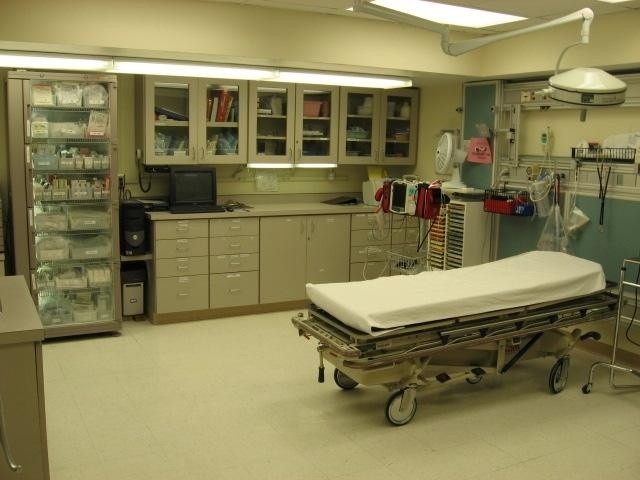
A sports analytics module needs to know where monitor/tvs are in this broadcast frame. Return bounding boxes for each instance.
[170,168,216,206]
[389,180,415,215]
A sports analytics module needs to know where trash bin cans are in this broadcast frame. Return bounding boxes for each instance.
[122,268,145,318]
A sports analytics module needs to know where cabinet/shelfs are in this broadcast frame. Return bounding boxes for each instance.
[351,215,420,281]
[4,70,122,339]
[260,213,349,314]
[144,218,260,325]
[135,74,419,165]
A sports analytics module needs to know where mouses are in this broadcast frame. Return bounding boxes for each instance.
[227,207,233,211]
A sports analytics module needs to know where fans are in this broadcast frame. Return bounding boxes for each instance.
[433,133,468,190]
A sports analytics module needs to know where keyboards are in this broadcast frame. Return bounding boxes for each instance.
[172,205,225,214]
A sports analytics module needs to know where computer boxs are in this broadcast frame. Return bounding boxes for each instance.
[119,199,146,256]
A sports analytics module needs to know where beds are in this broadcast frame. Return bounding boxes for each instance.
[290,253,626,426]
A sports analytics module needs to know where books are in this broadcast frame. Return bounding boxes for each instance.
[155,105,184,120]
[206,91,238,122]
